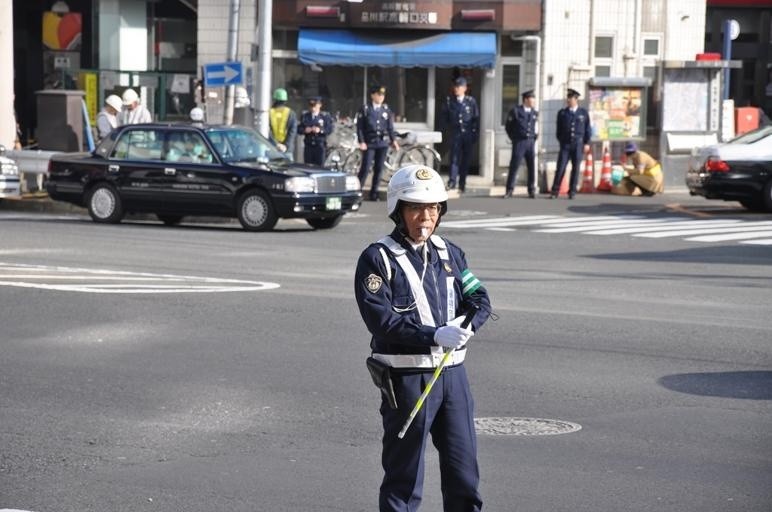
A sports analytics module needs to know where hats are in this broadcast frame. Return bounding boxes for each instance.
[43,122,364,232]
[0,155,22,201]
[686,122,772,213]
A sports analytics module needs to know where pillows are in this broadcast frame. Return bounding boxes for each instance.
[104,88,139,113]
[386,164,451,234]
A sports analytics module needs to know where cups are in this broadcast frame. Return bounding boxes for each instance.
[404,203,444,216]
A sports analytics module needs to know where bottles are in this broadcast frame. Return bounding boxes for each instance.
[625,143,637,154]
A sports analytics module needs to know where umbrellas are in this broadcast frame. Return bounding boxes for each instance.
[504,187,574,199]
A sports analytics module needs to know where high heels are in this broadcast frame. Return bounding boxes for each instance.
[557,169,569,196]
[595,147,615,191]
[578,150,597,194]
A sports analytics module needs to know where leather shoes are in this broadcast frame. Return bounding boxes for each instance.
[434,326,475,351]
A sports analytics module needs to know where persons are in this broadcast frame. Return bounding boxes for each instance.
[503,87,542,198]
[299,96,333,167]
[95,93,124,139]
[547,88,591,201]
[440,77,479,192]
[269,89,298,165]
[353,163,496,511]
[121,87,162,149]
[621,142,667,198]
[357,83,401,203]
